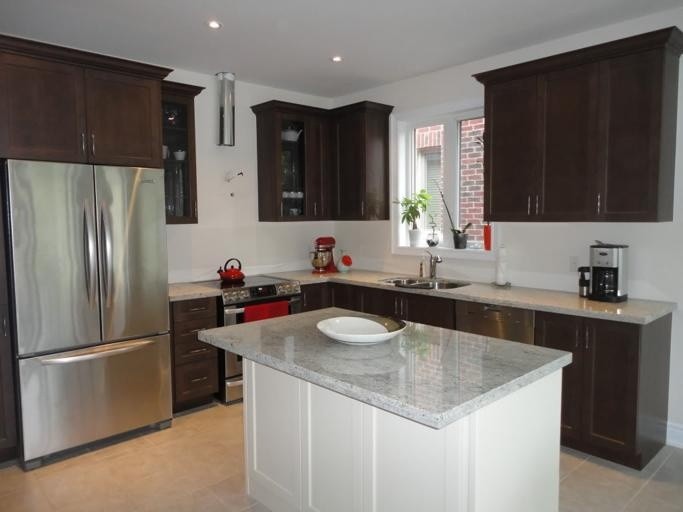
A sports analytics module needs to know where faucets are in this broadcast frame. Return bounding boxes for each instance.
[425,250,442,280]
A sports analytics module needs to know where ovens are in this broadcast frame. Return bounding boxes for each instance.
[219,294,301,404]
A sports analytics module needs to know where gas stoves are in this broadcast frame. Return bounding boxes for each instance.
[191,274,301,304]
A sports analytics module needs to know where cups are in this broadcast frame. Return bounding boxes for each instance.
[576,267,590,297]
[281,191,304,199]
[161,145,170,160]
[172,150,186,160]
[579,296,588,311]
[288,208,301,216]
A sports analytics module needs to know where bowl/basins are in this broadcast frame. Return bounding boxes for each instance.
[317,315,406,346]
[310,342,407,374]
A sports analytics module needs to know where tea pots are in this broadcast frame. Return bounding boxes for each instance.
[216,258,244,281]
[219,278,244,288]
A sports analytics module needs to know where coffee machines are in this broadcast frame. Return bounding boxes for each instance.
[587,240,629,304]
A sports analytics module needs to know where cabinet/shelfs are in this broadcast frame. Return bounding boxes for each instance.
[250,100,393,222]
[170,280,330,419]
[472,26,683,223]
[161,79,206,224]
[0,34,174,169]
[330,280,679,469]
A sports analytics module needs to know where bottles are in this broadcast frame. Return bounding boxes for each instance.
[483,221,490,251]
[335,248,350,273]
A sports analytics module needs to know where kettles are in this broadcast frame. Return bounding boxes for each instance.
[280,126,303,141]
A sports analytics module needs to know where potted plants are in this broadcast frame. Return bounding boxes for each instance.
[432,178,472,249]
[392,189,432,248]
[426,214,439,248]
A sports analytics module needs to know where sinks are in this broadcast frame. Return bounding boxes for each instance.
[377,276,426,287]
[409,279,473,290]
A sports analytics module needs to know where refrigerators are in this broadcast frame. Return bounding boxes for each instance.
[0,156,175,471]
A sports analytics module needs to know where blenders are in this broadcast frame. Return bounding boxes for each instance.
[307,236,338,273]
